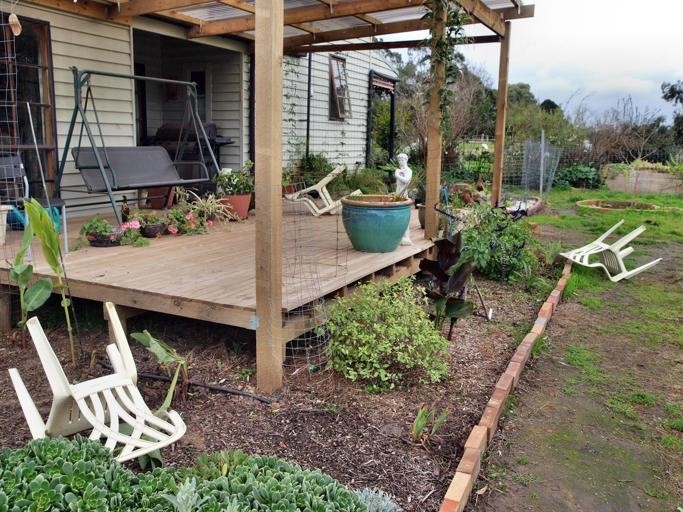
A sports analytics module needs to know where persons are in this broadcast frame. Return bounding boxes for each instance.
[394,153,413,198]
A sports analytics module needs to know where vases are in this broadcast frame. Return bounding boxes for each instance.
[220,191,251,220]
[341,193,412,254]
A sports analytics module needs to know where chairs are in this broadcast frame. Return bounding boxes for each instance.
[284,165,362,217]
[6,298,188,463]
[1,155,68,267]
[555,217,663,283]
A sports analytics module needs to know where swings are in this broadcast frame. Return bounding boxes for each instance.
[71,80,210,194]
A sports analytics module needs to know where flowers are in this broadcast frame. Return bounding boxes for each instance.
[69,199,215,252]
[214,161,256,195]
